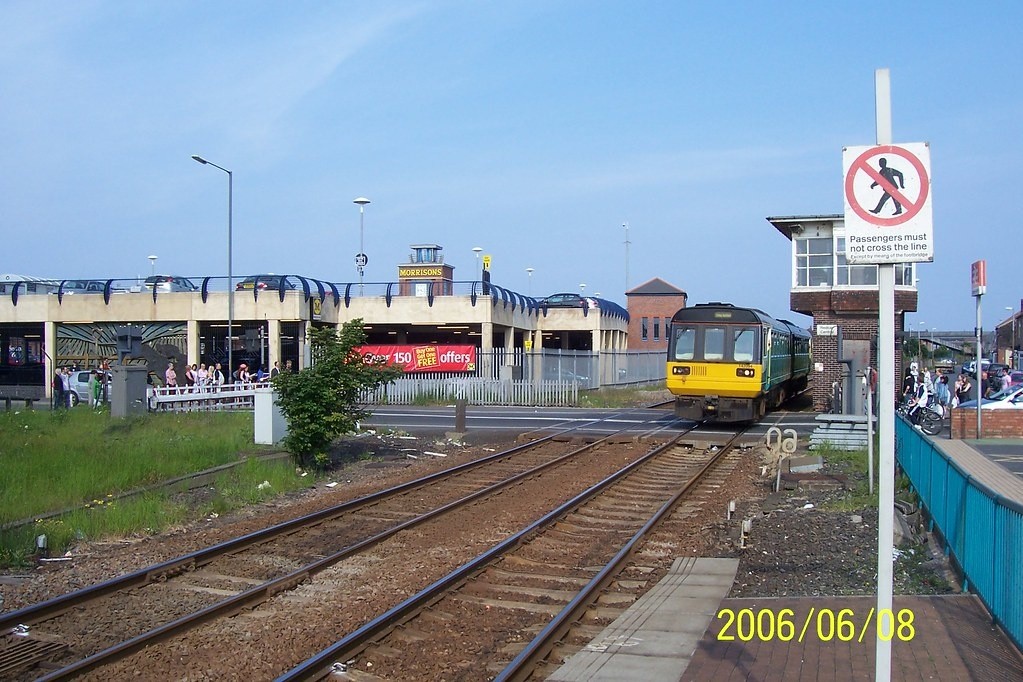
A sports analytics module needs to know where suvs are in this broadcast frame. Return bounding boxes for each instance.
[536,293,587,308]
[64,370,154,407]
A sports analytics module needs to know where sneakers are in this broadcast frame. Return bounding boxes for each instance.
[914,424,921,430]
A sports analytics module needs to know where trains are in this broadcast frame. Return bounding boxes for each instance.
[665,301,812,424]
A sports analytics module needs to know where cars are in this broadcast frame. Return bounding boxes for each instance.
[1007,370,1023,393]
[962,360,990,378]
[236,274,296,291]
[582,297,598,308]
[49,280,111,294]
[144,274,199,293]
[987,362,1009,381]
[957,383,1023,409]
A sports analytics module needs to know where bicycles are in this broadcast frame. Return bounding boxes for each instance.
[898,394,945,436]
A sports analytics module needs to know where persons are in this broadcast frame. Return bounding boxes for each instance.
[871,368,877,414]
[1001,369,1011,396]
[982,373,990,398]
[184,363,224,410]
[166,362,177,395]
[53,366,73,410]
[903,366,971,431]
[233,360,293,407]
[90,357,112,409]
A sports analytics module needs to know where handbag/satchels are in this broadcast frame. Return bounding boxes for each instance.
[951,397,959,409]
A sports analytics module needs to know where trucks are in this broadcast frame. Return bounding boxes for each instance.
[935,360,954,374]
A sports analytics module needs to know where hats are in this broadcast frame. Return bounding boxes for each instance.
[240,364,246,368]
[73,361,77,363]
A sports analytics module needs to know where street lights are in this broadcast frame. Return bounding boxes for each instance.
[190,155,234,403]
[580,284,586,297]
[472,246,482,295]
[353,197,370,296]
[931,328,937,358]
[919,322,925,362]
[1005,306,1015,351]
[526,267,534,297]
